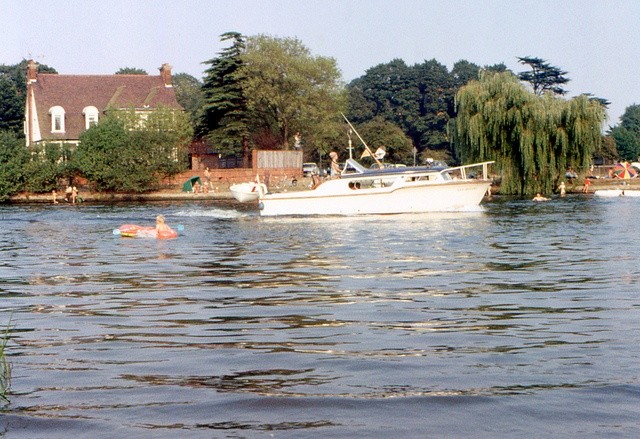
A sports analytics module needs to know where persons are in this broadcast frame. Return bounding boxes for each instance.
[294,129,302,149]
[608,168,621,179]
[633,170,640,178]
[349,183,355,190]
[65,185,80,204]
[192,167,212,196]
[154,214,171,234]
[636,171,640,178]
[356,183,360,189]
[329,152,342,176]
[583,178,589,194]
[587,169,598,178]
[532,193,549,201]
[52,190,58,204]
[564,167,578,179]
[559,181,565,195]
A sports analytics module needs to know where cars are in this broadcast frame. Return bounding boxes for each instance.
[468,172,501,187]
[302,163,320,176]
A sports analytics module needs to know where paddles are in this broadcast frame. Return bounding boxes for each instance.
[169,225,184,231]
[113,227,138,236]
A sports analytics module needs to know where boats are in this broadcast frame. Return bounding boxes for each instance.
[258,159,495,216]
[595,189,640,197]
[231,184,267,201]
